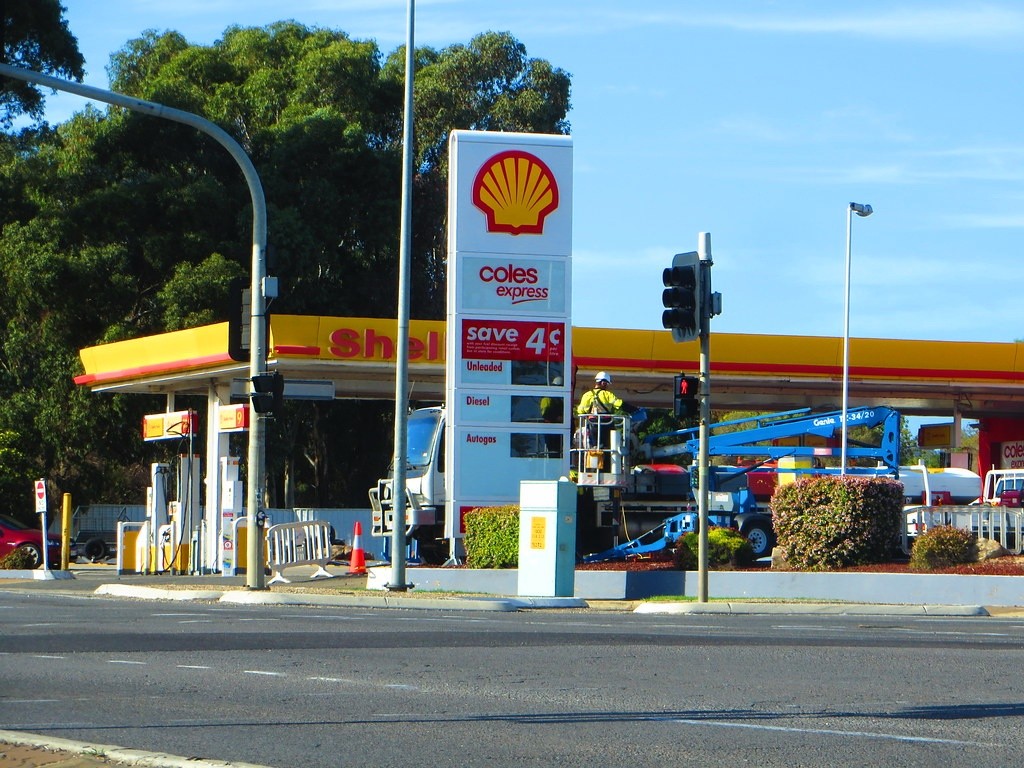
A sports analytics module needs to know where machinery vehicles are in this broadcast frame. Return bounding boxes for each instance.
[368,401,902,567]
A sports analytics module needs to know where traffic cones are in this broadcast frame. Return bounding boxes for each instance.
[344,521,369,574]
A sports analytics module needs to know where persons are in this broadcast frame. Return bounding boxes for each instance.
[577,371,640,472]
[540,377,564,458]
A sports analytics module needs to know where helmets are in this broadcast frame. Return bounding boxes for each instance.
[595,371,613,385]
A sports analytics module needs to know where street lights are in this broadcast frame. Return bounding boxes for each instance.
[841,201,874,477]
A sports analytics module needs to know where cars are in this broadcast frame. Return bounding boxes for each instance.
[0,514,78,570]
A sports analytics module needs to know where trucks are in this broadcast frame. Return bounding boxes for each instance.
[899,458,1024,553]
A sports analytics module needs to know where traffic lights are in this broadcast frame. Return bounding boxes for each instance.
[673,374,700,420]
[250,373,285,414]
[662,250,702,342]
